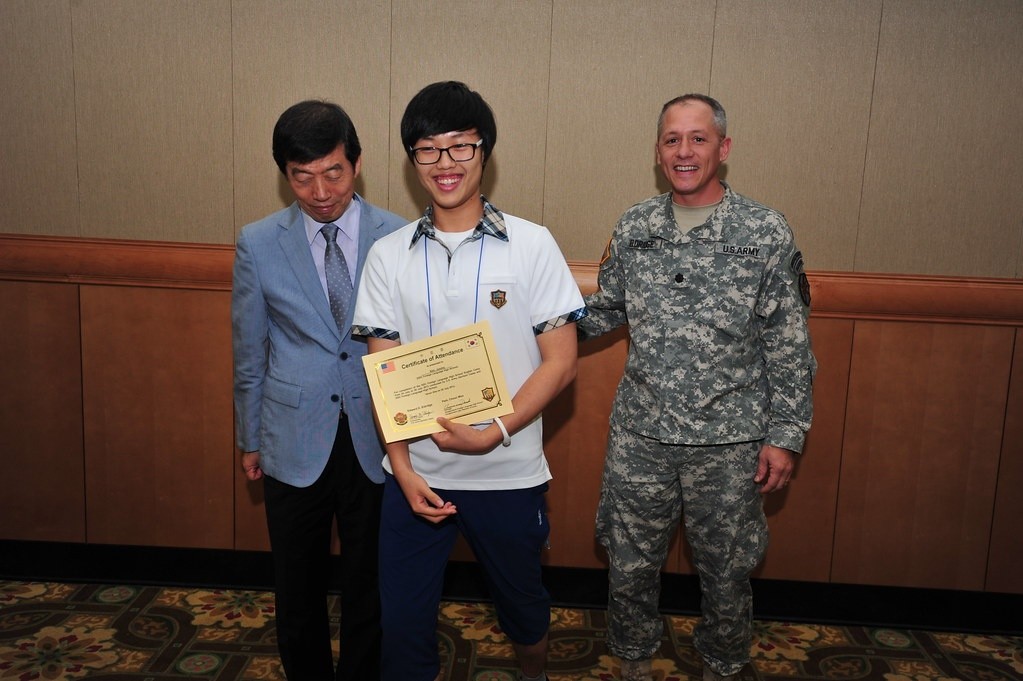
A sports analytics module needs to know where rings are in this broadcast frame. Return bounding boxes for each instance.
[786,480,789,483]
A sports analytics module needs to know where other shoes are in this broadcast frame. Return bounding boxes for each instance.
[620,656,652,681]
[703,664,735,681]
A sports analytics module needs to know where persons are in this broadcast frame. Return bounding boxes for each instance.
[575,95,814,681]
[233,103,413,681]
[348,80,589,681]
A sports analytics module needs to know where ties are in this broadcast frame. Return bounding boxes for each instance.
[320,224,353,335]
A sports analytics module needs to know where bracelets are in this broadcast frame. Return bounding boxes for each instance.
[493,417,511,447]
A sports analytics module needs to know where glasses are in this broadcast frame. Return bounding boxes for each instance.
[410,138,483,165]
